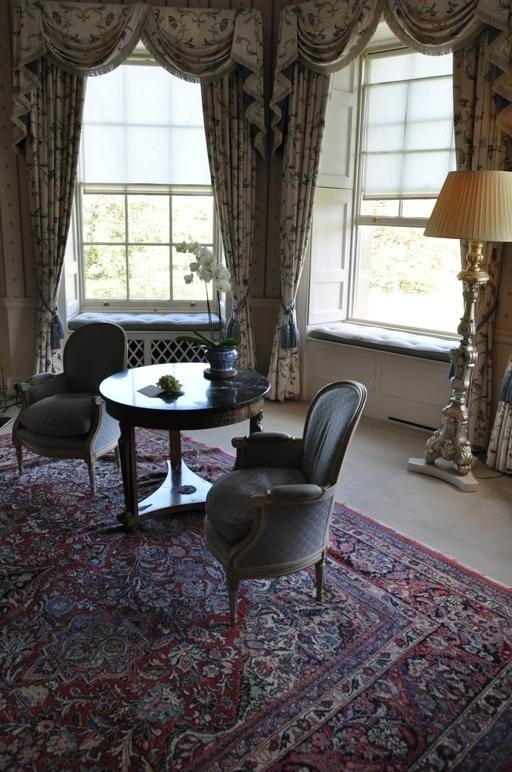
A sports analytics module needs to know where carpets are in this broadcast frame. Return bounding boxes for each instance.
[0,421,512,727]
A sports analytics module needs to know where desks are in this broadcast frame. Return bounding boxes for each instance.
[98,359,273,536]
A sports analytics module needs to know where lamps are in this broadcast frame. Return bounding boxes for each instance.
[408,167,512,494]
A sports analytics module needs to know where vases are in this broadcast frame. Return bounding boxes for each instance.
[203,344,240,376]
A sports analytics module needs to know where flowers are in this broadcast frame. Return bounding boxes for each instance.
[175,241,241,346]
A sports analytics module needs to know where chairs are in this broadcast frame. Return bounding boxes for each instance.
[10,321,129,497]
[202,377,372,625]
